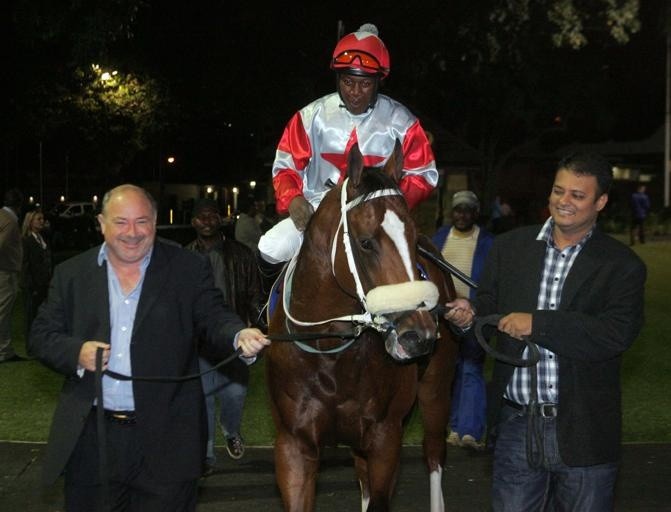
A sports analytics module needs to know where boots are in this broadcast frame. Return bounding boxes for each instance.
[259,252,285,301]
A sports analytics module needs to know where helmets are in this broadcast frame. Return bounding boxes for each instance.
[330,31,391,80]
[452,190,479,212]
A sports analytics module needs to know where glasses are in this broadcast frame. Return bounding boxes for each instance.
[332,49,379,70]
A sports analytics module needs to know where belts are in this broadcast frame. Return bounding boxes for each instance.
[105,410,135,424]
[505,400,556,418]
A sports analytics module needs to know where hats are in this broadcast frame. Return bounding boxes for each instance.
[190,197,221,216]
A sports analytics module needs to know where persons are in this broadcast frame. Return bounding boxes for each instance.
[182,198,261,477]
[26,182,272,512]
[2,190,552,360]
[443,144,649,511]
[416,190,496,452]
[631,183,651,245]
[256,30,440,321]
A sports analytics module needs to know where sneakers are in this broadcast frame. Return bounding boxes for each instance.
[203,455,218,478]
[447,432,462,449]
[217,420,245,460]
[460,435,485,452]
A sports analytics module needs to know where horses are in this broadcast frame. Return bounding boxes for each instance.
[264,137,460,512]
